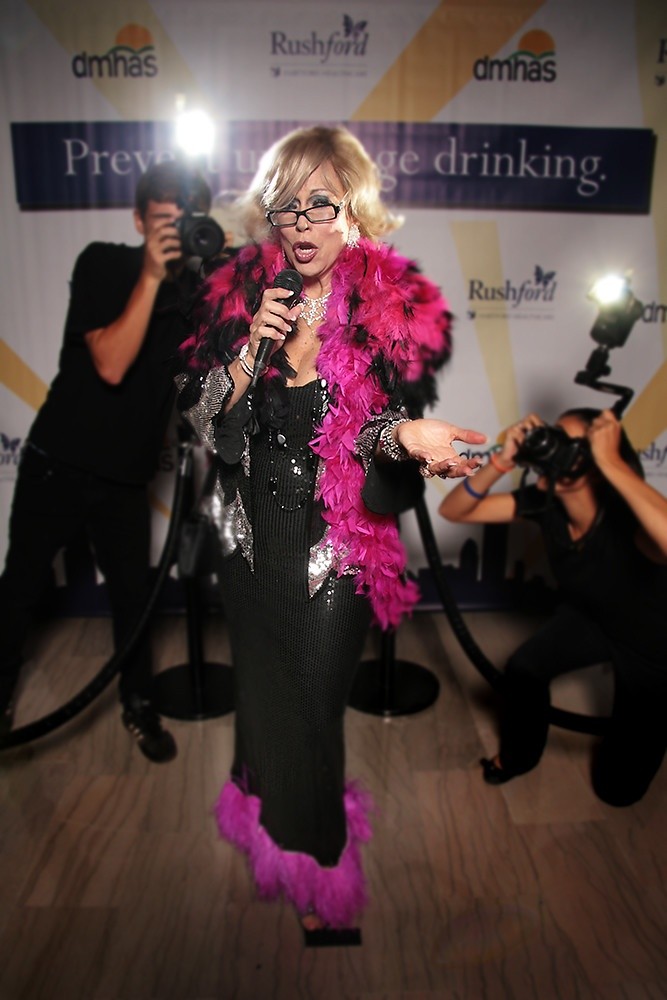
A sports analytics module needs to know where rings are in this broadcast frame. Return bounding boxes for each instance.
[419,463,445,479]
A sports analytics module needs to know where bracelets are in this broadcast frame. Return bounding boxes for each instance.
[462,476,489,499]
[239,342,270,379]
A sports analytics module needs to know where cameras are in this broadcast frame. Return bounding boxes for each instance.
[174,215,225,262]
[514,424,593,483]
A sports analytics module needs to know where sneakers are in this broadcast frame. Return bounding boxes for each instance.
[121,702,178,763]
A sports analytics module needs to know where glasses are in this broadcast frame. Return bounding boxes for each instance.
[264,188,352,226]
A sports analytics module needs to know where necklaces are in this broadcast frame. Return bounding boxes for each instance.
[299,293,331,328]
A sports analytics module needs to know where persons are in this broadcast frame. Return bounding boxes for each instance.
[1,164,237,762]
[172,121,486,932]
[438,408,667,807]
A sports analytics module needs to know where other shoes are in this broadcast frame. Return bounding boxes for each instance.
[484,755,540,786]
[296,902,361,947]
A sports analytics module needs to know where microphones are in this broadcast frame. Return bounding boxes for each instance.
[250,269,302,385]
